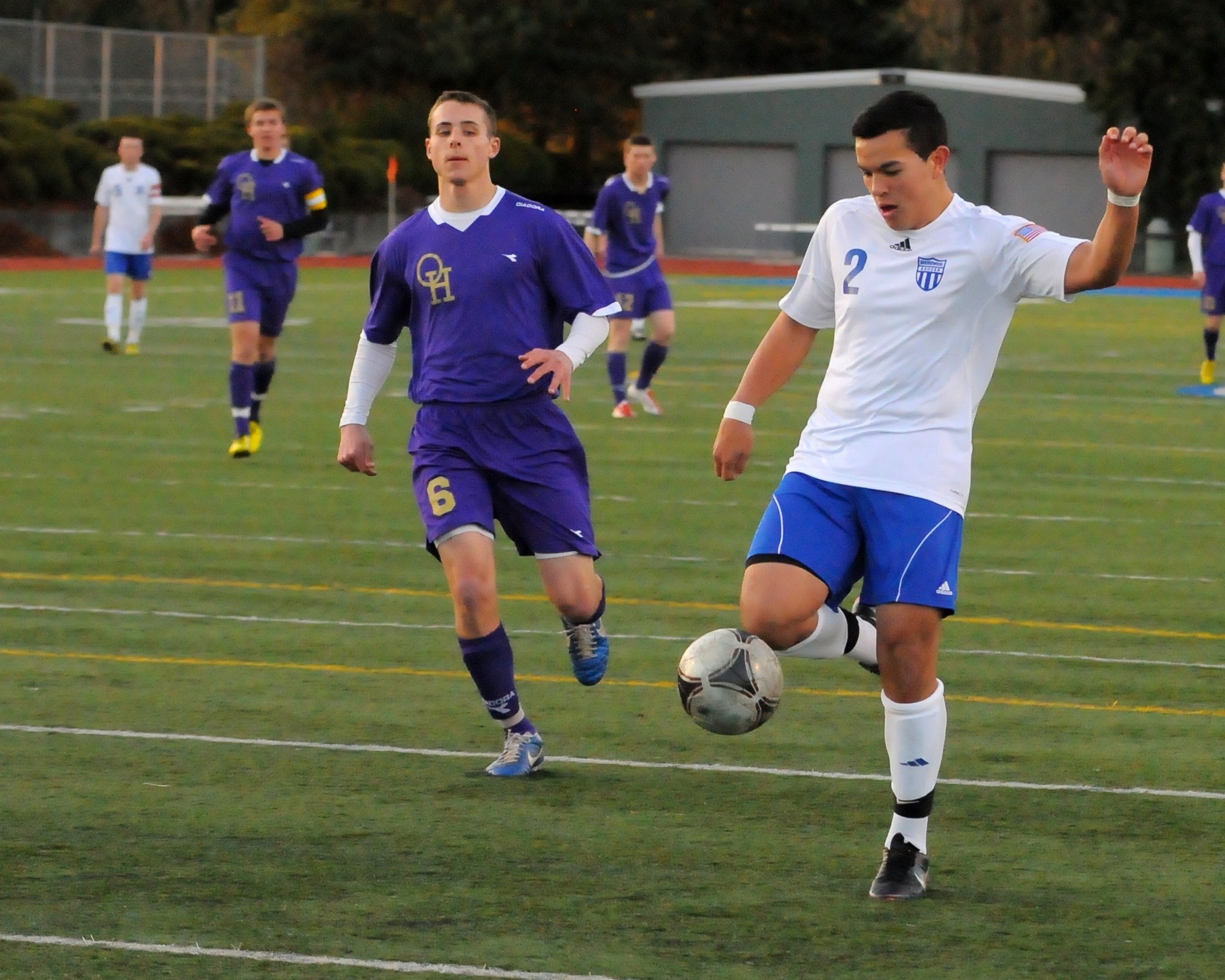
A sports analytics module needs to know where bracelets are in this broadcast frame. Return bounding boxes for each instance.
[1107,188,1141,207]
[723,399,756,425]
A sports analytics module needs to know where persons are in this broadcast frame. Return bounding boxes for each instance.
[713,87,1153,897]
[192,97,330,457]
[584,133,675,418]
[336,89,622,777]
[1186,160,1225,385]
[89,133,163,354]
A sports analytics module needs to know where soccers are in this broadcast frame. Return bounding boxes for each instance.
[675,626,784,735]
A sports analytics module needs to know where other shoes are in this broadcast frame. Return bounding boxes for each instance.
[125,343,138,356]
[229,435,251,457]
[1199,359,1216,384]
[102,338,120,356]
[248,421,261,453]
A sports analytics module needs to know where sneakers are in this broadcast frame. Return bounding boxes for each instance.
[484,728,545,779]
[851,594,881,677]
[627,385,661,415]
[611,400,636,419]
[868,832,930,900]
[560,612,609,686]
